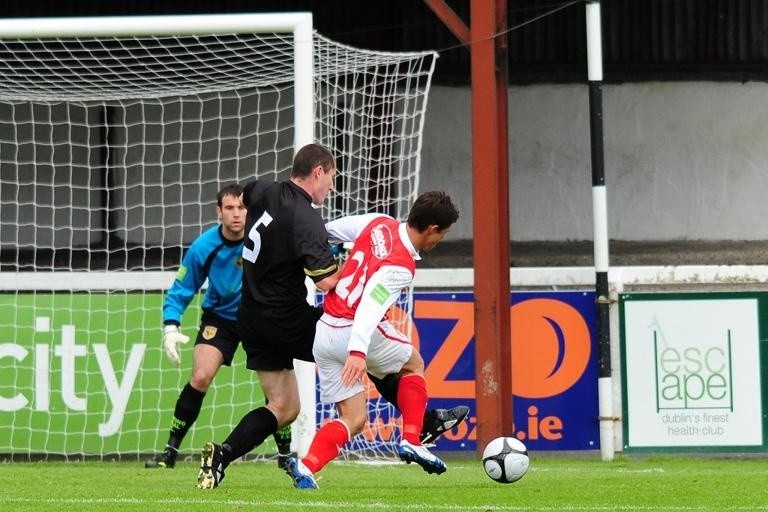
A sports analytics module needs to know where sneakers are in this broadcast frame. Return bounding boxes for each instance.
[196,442,225,489]
[278,451,318,489]
[400,405,468,474]
[145,448,177,469]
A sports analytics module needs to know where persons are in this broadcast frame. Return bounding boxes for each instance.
[285,191,459,489]
[145,184,297,468]
[197,144,470,490]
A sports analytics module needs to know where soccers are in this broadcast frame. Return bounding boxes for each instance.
[483,438,530,483]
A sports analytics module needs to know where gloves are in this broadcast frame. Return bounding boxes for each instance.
[162,325,190,364]
